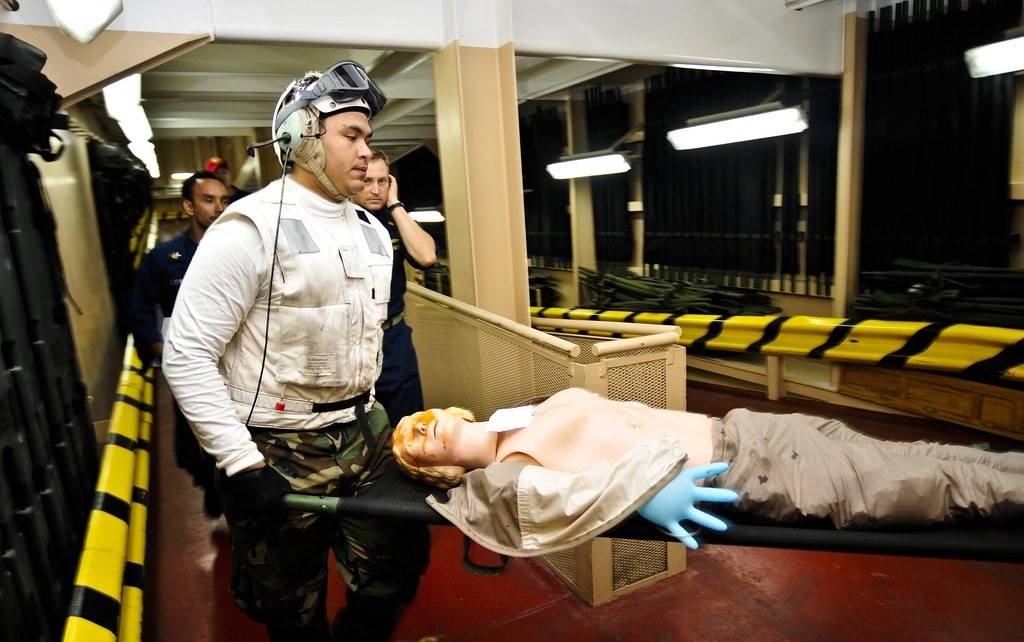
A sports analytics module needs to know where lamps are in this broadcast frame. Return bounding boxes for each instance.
[964,36,1024,81]
[406,205,446,229]
[546,144,644,181]
[666,99,810,152]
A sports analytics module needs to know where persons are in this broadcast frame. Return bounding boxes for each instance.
[347,147,438,429]
[204,154,252,205]
[162,61,432,642]
[391,388,1024,550]
[122,169,233,520]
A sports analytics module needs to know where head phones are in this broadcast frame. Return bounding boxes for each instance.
[274,109,345,201]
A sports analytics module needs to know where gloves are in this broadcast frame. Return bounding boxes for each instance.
[637,462,738,550]
[223,460,293,533]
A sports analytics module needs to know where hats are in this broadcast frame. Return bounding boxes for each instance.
[205,157,228,172]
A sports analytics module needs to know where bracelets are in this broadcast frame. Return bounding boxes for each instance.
[388,202,405,213]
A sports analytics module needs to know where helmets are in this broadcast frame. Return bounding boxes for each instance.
[272,76,371,167]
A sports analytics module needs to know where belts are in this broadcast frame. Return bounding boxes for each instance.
[382,310,404,330]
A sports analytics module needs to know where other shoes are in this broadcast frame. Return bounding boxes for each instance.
[203,477,223,519]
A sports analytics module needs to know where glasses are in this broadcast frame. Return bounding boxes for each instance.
[313,61,387,117]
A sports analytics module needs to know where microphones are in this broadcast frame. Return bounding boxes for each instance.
[247,134,287,158]
